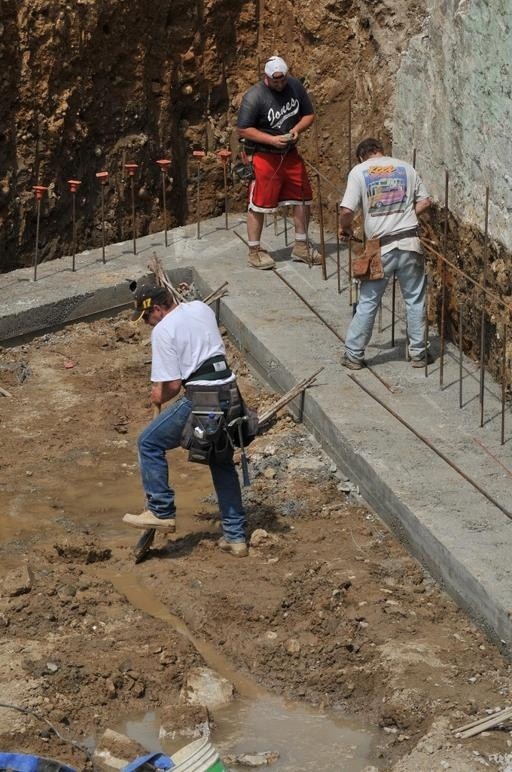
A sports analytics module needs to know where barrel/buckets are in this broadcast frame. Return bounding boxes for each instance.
[156,737,227,772]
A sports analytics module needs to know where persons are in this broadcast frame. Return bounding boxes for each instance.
[236,56,322,270]
[122,284,251,558]
[338,138,432,370]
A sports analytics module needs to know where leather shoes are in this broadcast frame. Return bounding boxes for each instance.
[122,510,176,534]
[216,535,248,558]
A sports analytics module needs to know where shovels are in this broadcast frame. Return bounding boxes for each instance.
[133,381,165,564]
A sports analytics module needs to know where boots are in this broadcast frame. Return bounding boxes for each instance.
[247,244,276,272]
[291,238,321,263]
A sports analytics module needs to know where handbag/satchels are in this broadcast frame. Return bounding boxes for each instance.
[180,388,259,465]
[231,143,256,187]
[351,238,384,282]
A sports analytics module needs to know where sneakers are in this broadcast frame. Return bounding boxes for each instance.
[339,356,362,370]
[410,349,432,368]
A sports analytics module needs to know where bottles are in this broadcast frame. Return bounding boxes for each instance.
[205,413,217,434]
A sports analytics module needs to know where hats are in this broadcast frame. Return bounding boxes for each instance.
[264,55,289,80]
[130,284,167,325]
